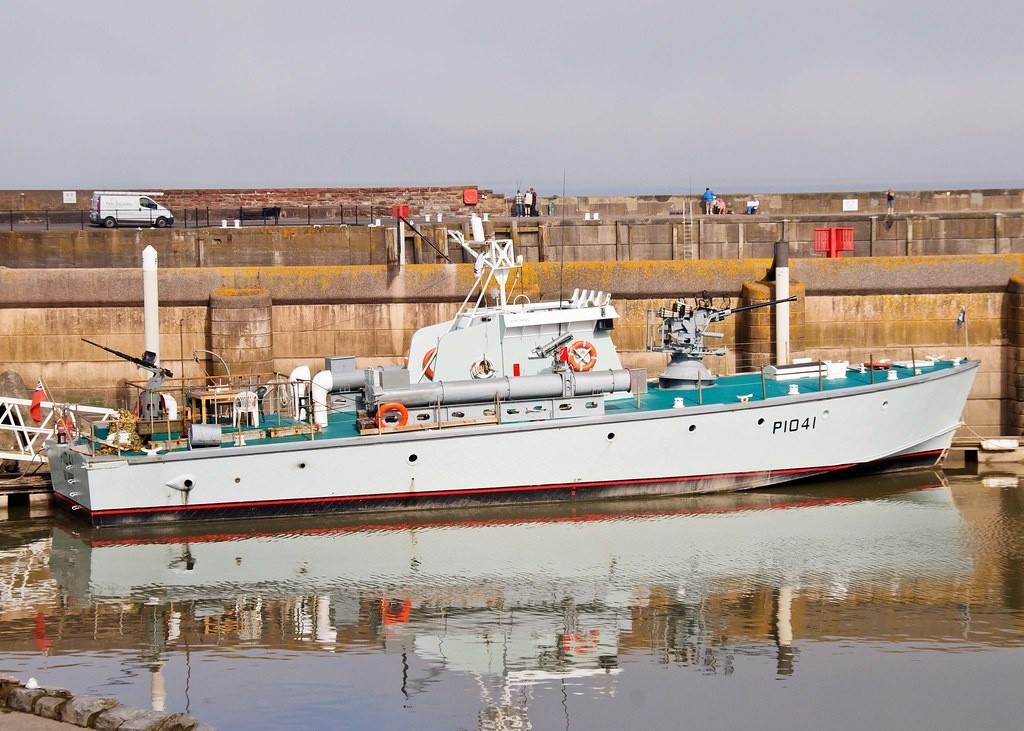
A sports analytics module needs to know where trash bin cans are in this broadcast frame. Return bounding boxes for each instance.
[548,203,555,216]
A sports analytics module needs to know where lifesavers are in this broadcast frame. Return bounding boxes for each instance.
[573,625,603,654]
[403,348,410,367]
[567,339,597,371]
[864,361,891,369]
[374,400,410,428]
[422,347,437,380]
[380,594,412,622]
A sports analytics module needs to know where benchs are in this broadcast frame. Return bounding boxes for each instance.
[240,205,279,224]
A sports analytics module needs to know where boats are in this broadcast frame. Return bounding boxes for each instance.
[46,229,981,528]
[49,468,973,731]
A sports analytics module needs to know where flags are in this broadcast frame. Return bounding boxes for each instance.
[29,378,48,424]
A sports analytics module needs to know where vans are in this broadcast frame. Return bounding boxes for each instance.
[89,191,174,227]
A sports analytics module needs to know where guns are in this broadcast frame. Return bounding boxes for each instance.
[80,338,174,377]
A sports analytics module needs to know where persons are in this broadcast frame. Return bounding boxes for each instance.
[745,197,759,215]
[514,188,537,218]
[703,187,726,216]
[885,188,895,215]
